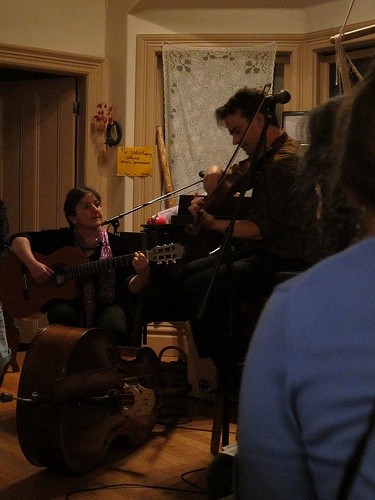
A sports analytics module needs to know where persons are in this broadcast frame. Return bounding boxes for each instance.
[7,188,151,344]
[159,89,323,402]
[238,65,375,500]
[0,200,9,249]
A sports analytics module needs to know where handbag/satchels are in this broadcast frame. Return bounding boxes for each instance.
[206,443,238,500]
[157,345,189,389]
[152,388,187,419]
[113,346,162,386]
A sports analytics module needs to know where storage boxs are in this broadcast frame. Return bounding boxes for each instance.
[142,321,218,400]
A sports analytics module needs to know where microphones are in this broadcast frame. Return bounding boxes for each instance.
[264,90,292,105]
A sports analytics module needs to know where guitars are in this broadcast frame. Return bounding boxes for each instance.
[0,240,188,319]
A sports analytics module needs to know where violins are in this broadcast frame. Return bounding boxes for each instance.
[185,145,274,237]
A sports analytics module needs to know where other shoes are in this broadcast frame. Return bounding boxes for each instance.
[0,389,14,402]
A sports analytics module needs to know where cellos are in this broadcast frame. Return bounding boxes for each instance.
[1,323,190,474]
